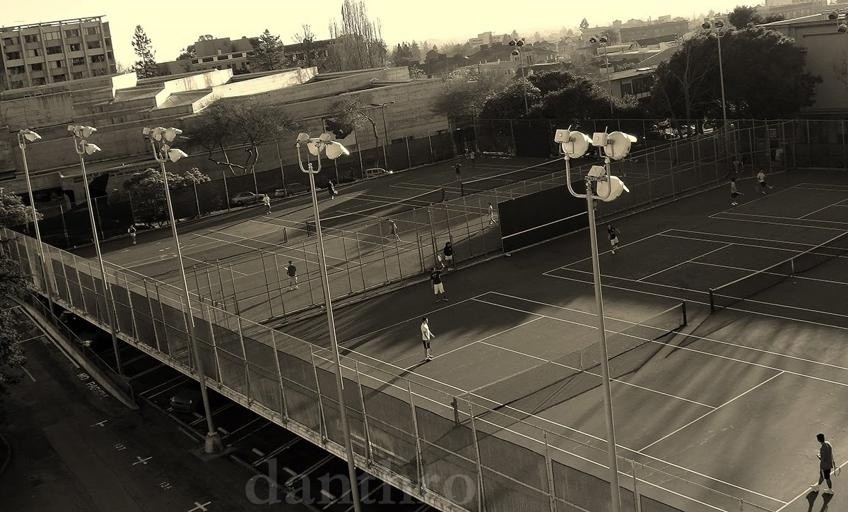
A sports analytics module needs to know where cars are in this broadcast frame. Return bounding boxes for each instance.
[275,183,310,197]
[231,191,265,207]
[170,389,201,413]
[365,168,385,178]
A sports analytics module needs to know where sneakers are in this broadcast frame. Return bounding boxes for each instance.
[811,484,834,494]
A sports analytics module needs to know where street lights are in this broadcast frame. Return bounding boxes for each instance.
[16,128,55,313]
[829,11,848,33]
[702,20,735,169]
[382,101,395,170]
[508,39,532,133]
[142,126,227,454]
[67,125,126,378]
[553,124,637,512]
[589,34,615,118]
[296,131,360,510]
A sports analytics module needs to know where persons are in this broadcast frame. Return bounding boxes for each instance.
[385,218,401,242]
[757,168,776,196]
[283,226,289,244]
[444,242,455,271]
[455,161,463,181]
[607,222,622,254]
[730,177,739,205]
[420,315,435,360]
[441,189,448,203]
[306,222,311,237]
[810,434,836,494]
[287,261,298,291]
[262,193,272,215]
[127,224,137,246]
[487,202,497,225]
[463,146,482,163]
[459,182,464,198]
[328,179,337,200]
[431,267,448,303]
